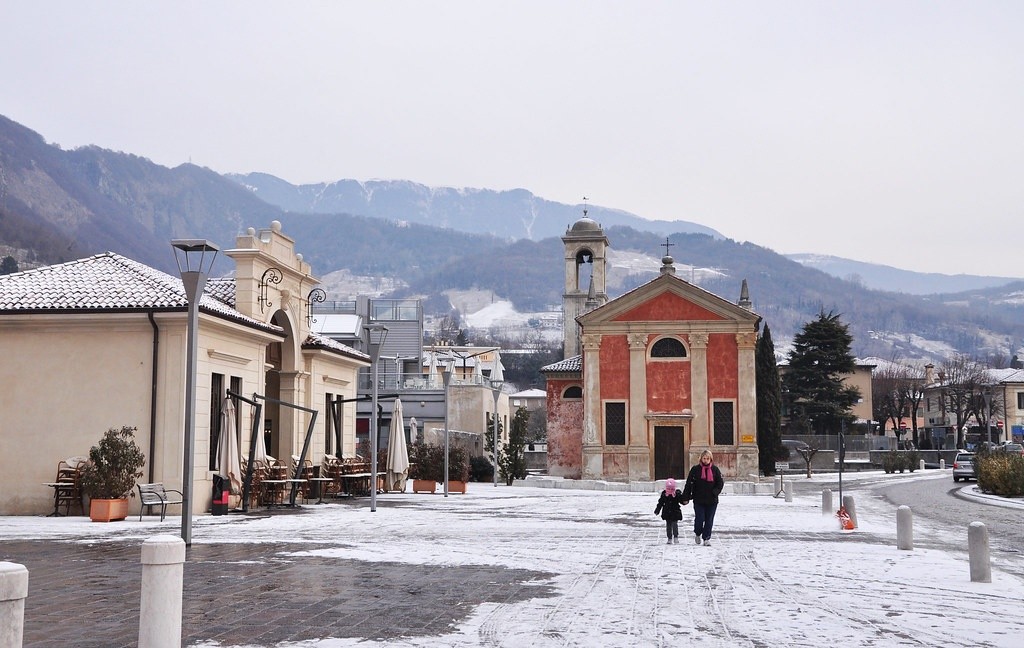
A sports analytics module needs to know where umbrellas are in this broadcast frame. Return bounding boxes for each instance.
[386,398,409,492]
[429,351,437,382]
[490,350,505,381]
[249,397,268,468]
[409,416,420,459]
[445,349,456,373]
[215,397,242,494]
[473,354,484,384]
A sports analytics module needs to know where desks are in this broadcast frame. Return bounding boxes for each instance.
[363,473,378,494]
[339,474,364,500]
[309,478,334,504]
[43,482,106,517]
[260,480,286,509]
[285,478,308,508]
[376,472,386,493]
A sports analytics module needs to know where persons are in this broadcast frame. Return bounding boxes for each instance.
[682,450,724,546]
[904,437,911,450]
[654,478,688,544]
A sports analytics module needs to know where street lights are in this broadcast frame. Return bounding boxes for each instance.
[168,238,218,546]
[983,389,992,451]
[437,357,456,497]
[362,322,389,511]
[490,380,504,487]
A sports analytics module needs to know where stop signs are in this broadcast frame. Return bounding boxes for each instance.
[998,422,1003,428]
[900,422,907,429]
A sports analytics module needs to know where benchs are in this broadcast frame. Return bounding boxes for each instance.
[136,483,182,522]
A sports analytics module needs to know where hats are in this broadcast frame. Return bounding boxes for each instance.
[665,477,676,497]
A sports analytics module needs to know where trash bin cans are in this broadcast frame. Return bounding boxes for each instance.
[211,473,230,515]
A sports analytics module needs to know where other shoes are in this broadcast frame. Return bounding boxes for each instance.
[695,535,700,544]
[674,538,679,543]
[667,538,672,544]
[703,540,710,546]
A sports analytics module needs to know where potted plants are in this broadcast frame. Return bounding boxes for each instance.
[442,443,470,494]
[411,436,443,493]
[77,426,145,522]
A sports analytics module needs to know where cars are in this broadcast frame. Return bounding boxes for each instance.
[983,442,998,450]
[1004,444,1024,457]
[953,452,977,481]
[997,441,1012,447]
[966,443,973,449]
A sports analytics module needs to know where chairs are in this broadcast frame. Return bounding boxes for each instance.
[403,377,473,391]
[53,455,92,516]
[238,454,382,508]
